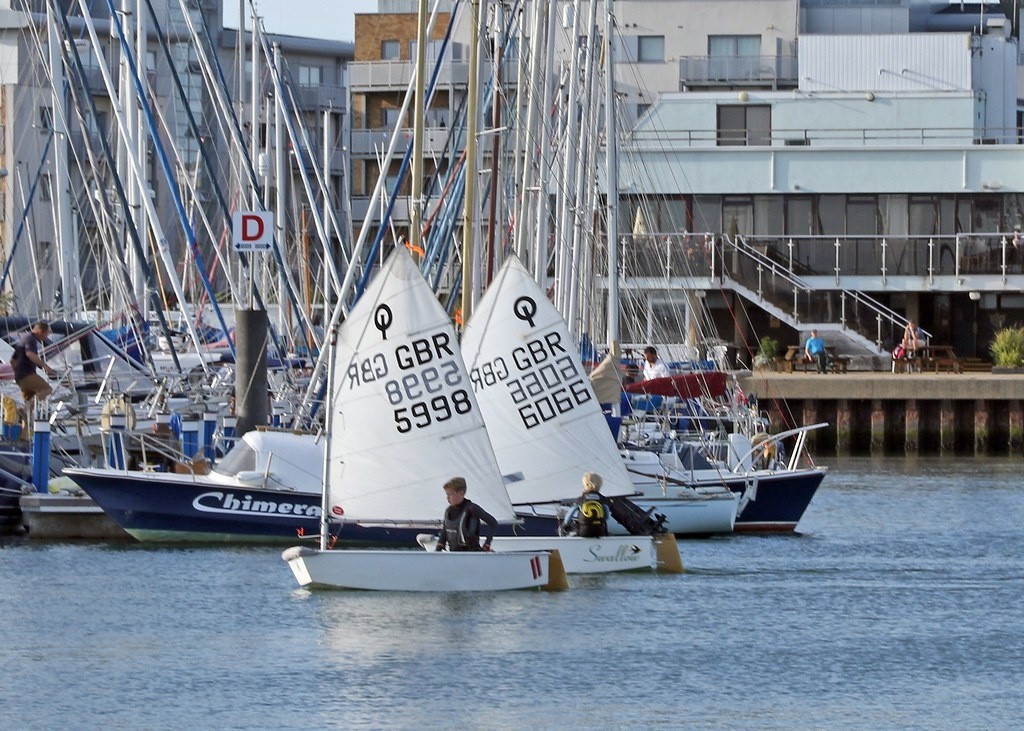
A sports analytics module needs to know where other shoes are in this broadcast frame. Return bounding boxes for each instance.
[817,369,821,374]
[821,368,827,374]
[15,406,28,423]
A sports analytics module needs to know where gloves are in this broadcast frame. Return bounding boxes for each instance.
[482,544,490,551]
[436,545,446,551]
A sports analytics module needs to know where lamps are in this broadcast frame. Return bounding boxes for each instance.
[739,91,749,102]
[969,291,981,300]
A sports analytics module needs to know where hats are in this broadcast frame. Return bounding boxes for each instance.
[811,329,817,333]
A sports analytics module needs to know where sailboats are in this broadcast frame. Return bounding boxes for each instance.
[458,248,683,574]
[0,0,830,547]
[280,234,570,592]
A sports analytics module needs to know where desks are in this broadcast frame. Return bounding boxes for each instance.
[785,345,836,372]
[904,345,957,374]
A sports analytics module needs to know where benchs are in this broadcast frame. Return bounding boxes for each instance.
[892,357,964,374]
[791,357,849,374]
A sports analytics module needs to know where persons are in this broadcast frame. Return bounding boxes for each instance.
[701,235,712,276]
[434,477,497,552]
[10,321,57,415]
[902,319,926,359]
[805,329,828,374]
[621,364,640,393]
[1011,230,1024,274]
[558,473,611,537]
[643,235,698,277]
[641,346,670,381]
